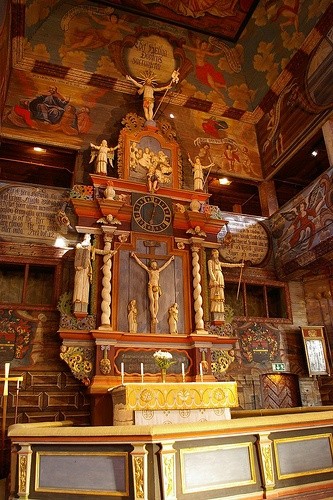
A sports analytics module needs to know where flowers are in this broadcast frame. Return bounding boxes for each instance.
[155,351,174,370]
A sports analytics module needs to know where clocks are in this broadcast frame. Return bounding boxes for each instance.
[132,197,171,233]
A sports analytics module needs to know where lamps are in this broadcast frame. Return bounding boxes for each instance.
[3,362,10,396]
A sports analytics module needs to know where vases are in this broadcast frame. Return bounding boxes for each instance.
[161,370,168,383]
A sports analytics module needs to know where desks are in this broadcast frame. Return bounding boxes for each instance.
[108,381,239,426]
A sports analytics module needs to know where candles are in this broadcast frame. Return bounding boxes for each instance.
[200,363,202,375]
[182,362,185,375]
[120,363,125,375]
[141,363,143,375]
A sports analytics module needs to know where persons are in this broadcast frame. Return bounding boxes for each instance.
[71,232,118,304]
[130,252,176,324]
[168,302,180,335]
[143,160,159,192]
[208,248,245,313]
[126,74,173,121]
[186,156,215,192]
[127,300,138,334]
[88,139,121,175]
